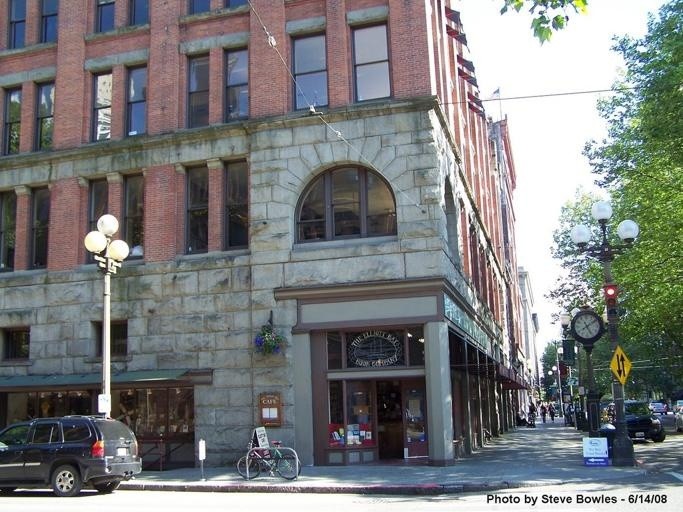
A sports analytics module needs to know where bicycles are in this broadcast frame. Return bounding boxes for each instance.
[237,439,301,480]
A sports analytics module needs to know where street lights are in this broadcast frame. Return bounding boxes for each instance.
[547,346,580,419]
[569,198,642,466]
[561,310,610,432]
[84,213,131,416]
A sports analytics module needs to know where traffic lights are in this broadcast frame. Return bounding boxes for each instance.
[603,286,620,319]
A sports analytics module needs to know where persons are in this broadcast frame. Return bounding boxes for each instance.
[529,402,535,427]
[547,403,555,422]
[540,403,546,422]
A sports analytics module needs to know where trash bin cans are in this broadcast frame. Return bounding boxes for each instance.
[597,424,616,457]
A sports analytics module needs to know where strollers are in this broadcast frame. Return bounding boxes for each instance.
[526,413,536,428]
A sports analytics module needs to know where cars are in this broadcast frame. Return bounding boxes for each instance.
[674,402,683,434]
[649,400,667,415]
[623,398,666,442]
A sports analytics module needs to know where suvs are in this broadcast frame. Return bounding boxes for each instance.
[0,410,143,496]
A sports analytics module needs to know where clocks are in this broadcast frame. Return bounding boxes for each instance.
[571,310,604,342]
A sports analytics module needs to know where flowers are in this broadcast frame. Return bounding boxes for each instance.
[253,324,285,355]
